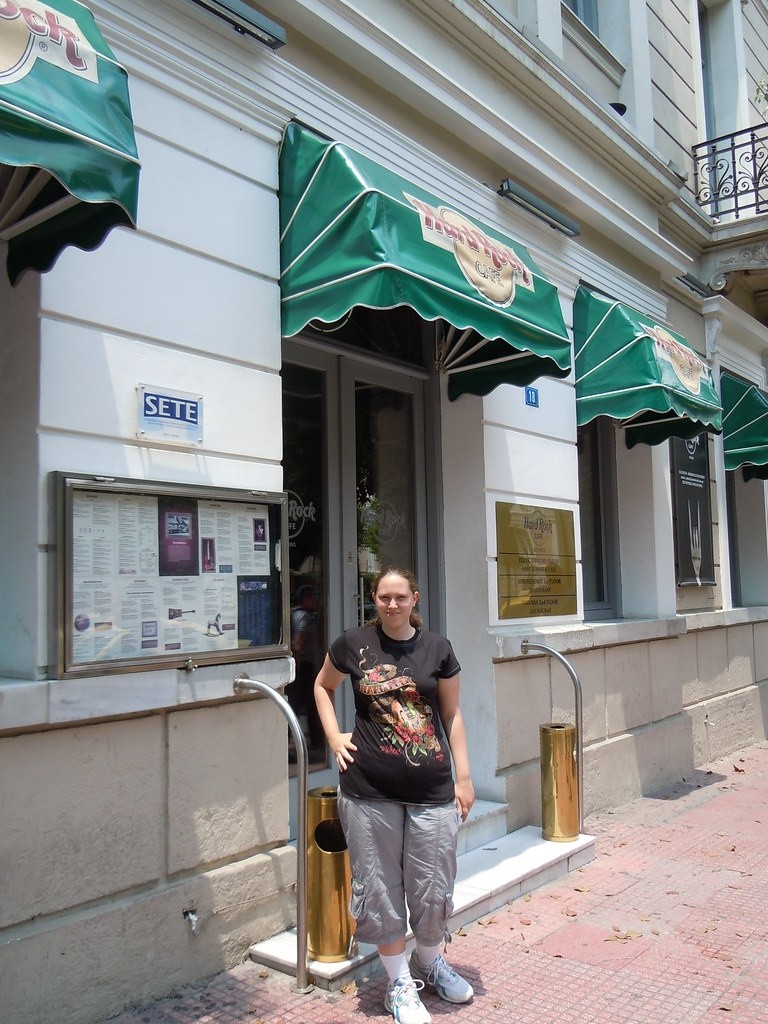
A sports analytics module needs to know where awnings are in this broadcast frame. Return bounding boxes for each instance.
[0,0,142,286]
[720,371,768,482]
[278,121,571,402]
[573,285,723,449]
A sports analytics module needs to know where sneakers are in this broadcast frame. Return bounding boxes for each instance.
[384,974,432,1024]
[409,948,474,1003]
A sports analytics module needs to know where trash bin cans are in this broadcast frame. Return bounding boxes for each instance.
[538,722,582,842]
[304,787,359,963]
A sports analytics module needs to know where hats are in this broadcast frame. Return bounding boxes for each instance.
[295,585,320,601]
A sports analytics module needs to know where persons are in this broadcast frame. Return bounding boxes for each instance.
[314,567,475,1024]
[291,585,325,751]
